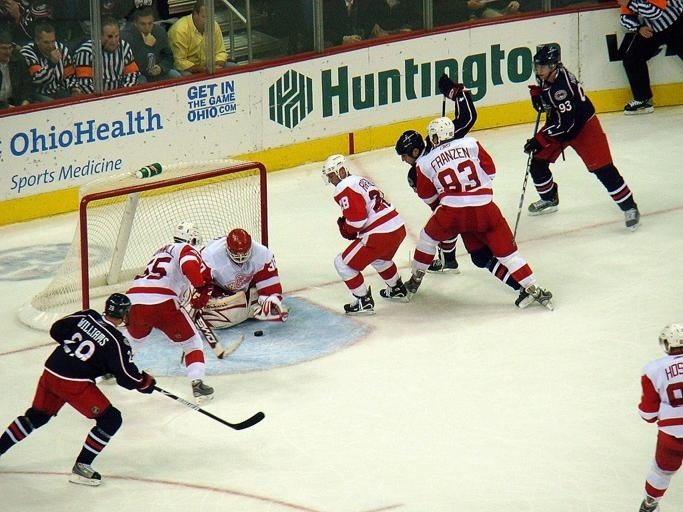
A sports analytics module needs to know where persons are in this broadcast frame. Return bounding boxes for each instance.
[398,118,552,306]
[295,0,536,48]
[192,227,283,315]
[524,44,638,227]
[322,154,406,310]
[112,219,208,385]
[396,77,535,303]
[639,324,681,507]
[0,293,153,482]
[1,1,226,106]
[618,0,682,110]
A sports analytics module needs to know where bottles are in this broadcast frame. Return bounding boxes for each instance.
[135,162,162,179]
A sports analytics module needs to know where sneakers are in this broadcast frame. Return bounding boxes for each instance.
[72,462,101,479]
[192,379,214,397]
[624,99,652,111]
[626,203,640,226]
[639,497,658,512]
[344,259,552,312]
[528,195,559,212]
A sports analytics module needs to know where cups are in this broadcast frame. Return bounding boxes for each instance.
[145,53,155,73]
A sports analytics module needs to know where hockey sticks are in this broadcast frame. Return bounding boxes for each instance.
[153,386,264,430]
[183,300,244,359]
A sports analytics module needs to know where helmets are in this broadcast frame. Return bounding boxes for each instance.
[102,293,131,327]
[395,130,425,160]
[227,229,252,264]
[533,43,560,76]
[658,322,683,354]
[321,154,350,186]
[428,116,455,147]
[174,221,203,249]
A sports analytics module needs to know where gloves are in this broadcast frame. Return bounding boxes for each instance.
[337,216,357,240]
[190,287,209,309]
[524,137,544,154]
[528,85,542,97]
[137,371,156,394]
[438,73,463,100]
